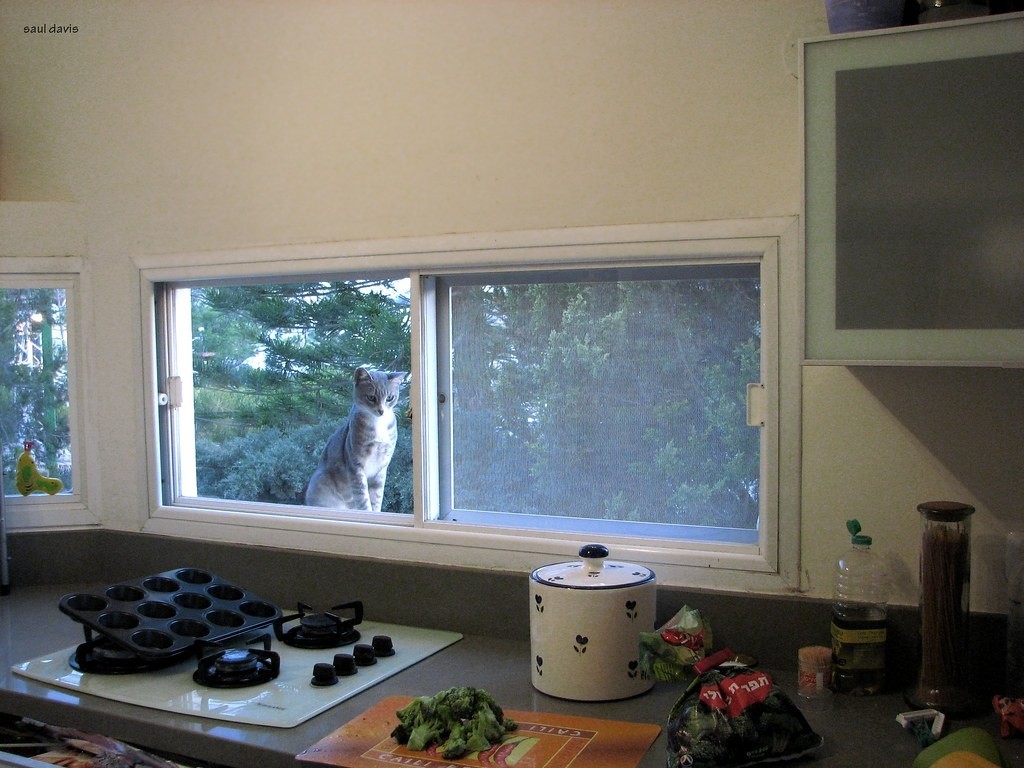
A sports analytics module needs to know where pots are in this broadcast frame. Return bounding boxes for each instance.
[529,545,656,701]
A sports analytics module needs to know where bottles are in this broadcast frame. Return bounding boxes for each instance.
[917,501,975,708]
[831,519,886,692]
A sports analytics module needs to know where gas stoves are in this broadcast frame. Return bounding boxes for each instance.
[12,606,463,728]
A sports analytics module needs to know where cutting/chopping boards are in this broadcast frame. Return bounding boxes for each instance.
[295,696,662,768]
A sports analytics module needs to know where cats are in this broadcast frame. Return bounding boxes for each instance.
[305,366,409,511]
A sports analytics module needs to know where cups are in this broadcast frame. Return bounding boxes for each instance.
[798,645,831,712]
